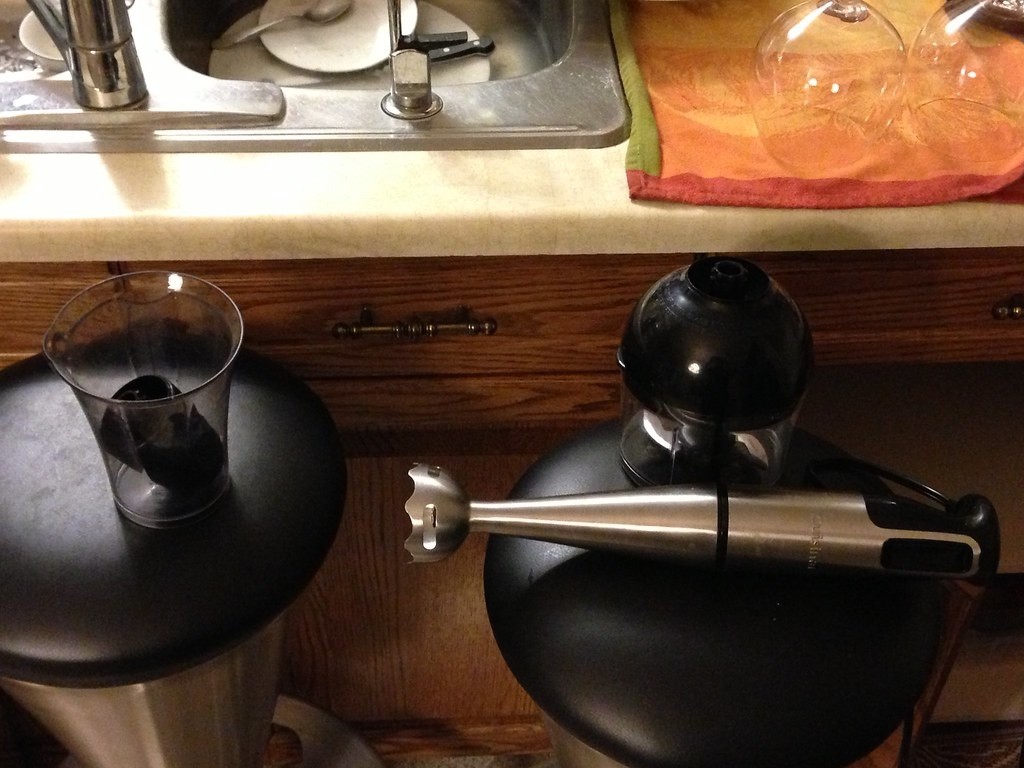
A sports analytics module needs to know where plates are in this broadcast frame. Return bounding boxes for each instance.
[208,0,491,89]
[258,0,417,73]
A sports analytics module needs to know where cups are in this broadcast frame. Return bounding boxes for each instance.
[44,269,245,528]
[748,2,907,178]
[909,1,1023,182]
[18,5,76,73]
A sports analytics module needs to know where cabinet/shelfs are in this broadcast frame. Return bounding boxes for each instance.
[0,246,1024,768]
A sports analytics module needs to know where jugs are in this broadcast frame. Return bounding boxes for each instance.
[23,0,148,108]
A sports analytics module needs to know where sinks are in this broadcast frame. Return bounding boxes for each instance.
[156,0,634,152]
[0,0,86,156]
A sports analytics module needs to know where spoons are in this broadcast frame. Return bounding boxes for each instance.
[212,0,352,53]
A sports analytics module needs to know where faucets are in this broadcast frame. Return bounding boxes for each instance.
[382,0,470,123]
[24,0,152,112]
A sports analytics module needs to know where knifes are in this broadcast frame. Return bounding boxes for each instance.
[274,34,495,87]
